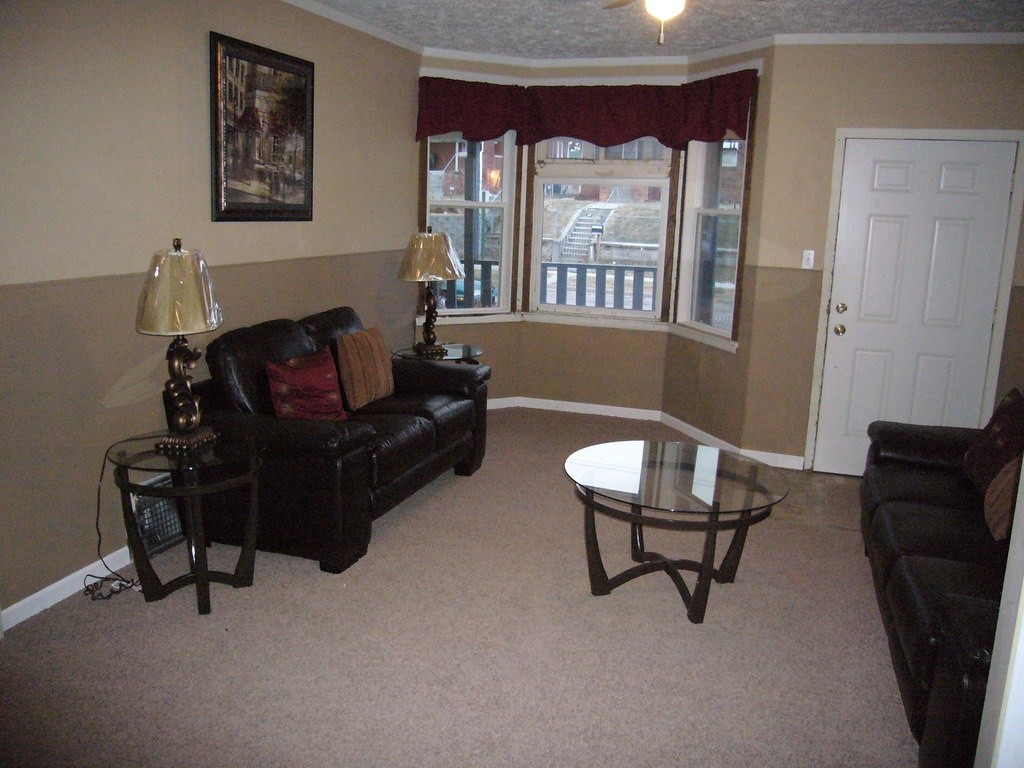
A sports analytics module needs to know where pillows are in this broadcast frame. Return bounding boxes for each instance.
[334,326,394,411]
[265,344,351,421]
[984,456,1023,541]
[961,387,1024,495]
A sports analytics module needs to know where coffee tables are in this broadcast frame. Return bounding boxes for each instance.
[564,438,789,625]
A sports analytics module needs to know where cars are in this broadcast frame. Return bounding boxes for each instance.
[424,278,497,309]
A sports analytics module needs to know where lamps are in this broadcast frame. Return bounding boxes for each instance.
[135,238,223,451]
[397,225,466,357]
[645,0,685,45]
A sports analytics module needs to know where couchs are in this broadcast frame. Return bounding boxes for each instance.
[163,308,492,574]
[859,421,1012,768]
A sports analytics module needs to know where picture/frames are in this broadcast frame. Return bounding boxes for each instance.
[208,30,315,223]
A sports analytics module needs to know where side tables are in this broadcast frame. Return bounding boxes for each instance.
[390,342,485,365]
[105,420,268,614]
